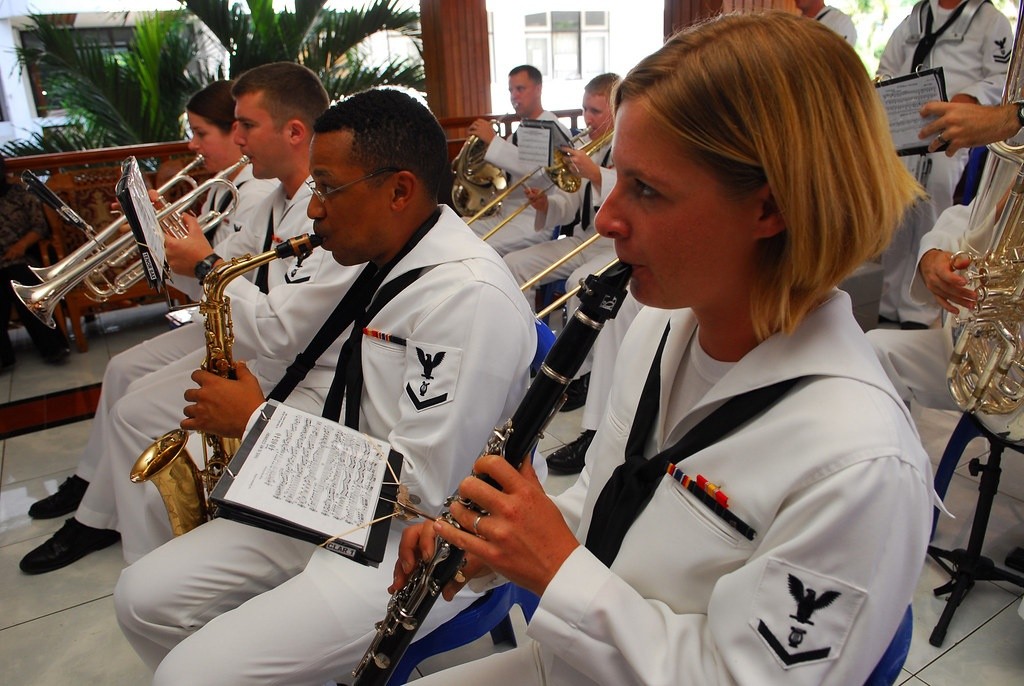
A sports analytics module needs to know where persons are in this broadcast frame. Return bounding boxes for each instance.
[867,101,1023,571]
[385,12,956,686]
[113,90,550,686]
[460,65,573,258]
[878,0,1014,327]
[502,73,643,474]
[797,0,857,47]
[0,156,71,371]
[18,61,368,574]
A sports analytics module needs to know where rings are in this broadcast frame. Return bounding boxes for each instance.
[938,132,947,144]
[473,514,489,539]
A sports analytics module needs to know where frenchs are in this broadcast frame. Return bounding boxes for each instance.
[449,105,517,219]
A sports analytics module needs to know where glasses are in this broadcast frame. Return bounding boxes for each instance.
[306,166,402,204]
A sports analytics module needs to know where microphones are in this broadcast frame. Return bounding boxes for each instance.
[20,169,96,236]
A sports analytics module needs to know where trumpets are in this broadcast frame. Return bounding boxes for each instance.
[29,154,203,277]
[13,153,255,331]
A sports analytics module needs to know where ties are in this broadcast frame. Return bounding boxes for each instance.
[910,0,970,71]
[251,210,274,293]
[585,317,825,569]
[321,264,444,431]
[505,129,519,187]
[817,9,830,21]
[581,149,611,231]
[203,180,246,242]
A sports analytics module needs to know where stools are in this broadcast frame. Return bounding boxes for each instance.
[929,411,1024,542]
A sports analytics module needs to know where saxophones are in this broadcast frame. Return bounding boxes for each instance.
[945,0,1023,415]
[133,234,322,538]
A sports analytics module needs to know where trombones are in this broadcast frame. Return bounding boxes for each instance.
[465,122,620,241]
[519,230,617,319]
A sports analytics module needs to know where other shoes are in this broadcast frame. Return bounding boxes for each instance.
[900,318,928,329]
[875,314,899,325]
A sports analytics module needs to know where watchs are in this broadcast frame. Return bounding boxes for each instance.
[195,253,223,286]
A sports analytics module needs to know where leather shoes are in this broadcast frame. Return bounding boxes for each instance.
[20,515,121,574]
[29,476,85,518]
[547,429,597,474]
[559,372,592,412]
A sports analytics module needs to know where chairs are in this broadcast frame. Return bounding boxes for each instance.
[47,156,155,355]
[6,176,70,335]
[154,159,216,304]
[389,322,557,686]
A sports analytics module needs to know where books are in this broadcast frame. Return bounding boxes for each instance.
[518,118,576,167]
[873,64,948,156]
[209,398,404,569]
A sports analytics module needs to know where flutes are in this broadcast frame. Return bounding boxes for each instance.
[339,262,632,686]
